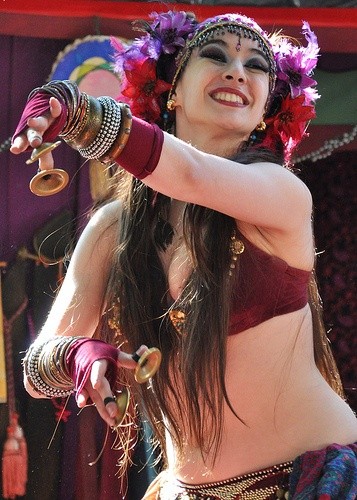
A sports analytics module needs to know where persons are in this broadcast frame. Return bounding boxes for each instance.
[10,10,357,500]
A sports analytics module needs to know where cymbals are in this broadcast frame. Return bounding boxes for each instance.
[134,347,161,384]
[29,168,70,197]
[109,388,131,432]
[26,141,62,166]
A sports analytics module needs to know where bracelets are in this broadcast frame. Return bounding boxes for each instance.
[58,77,133,164]
[28,336,76,398]
